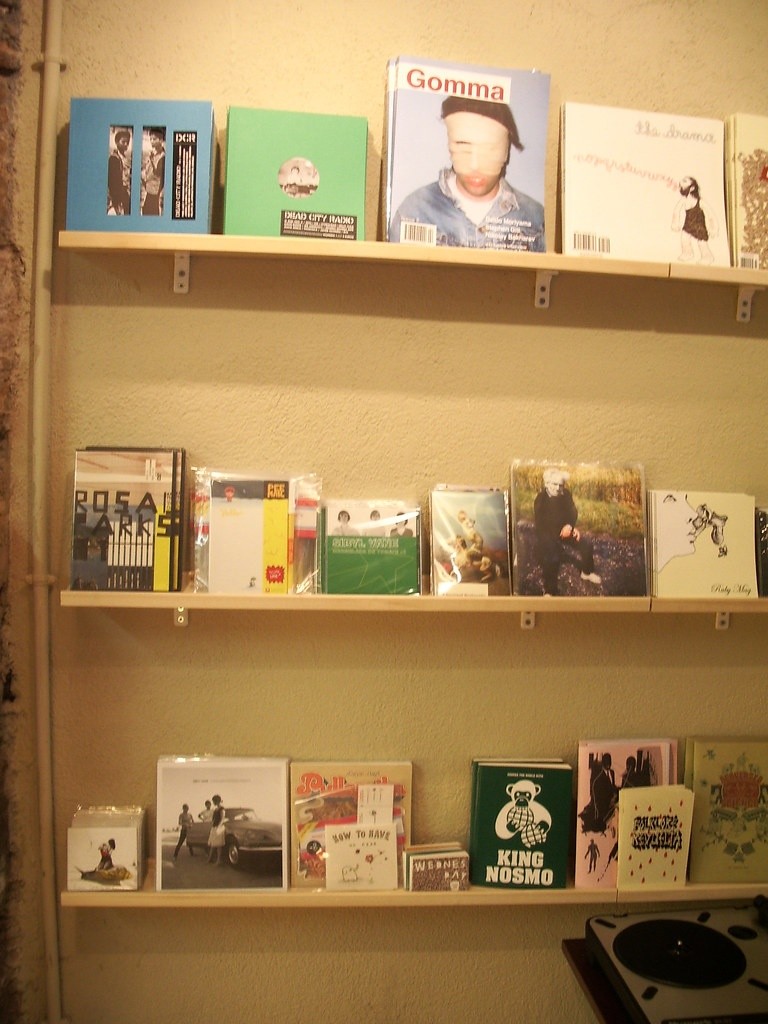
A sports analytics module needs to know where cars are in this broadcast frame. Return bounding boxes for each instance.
[186,805,283,866]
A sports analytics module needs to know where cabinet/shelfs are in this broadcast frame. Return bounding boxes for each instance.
[57,232,767,909]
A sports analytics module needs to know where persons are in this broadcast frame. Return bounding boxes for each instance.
[391,94,547,252]
[621,753,638,786]
[108,125,165,216]
[173,795,228,868]
[533,470,602,598]
[330,512,416,539]
[593,752,613,824]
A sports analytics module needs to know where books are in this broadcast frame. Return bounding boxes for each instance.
[558,102,768,270]
[646,490,761,600]
[381,55,550,253]
[289,759,468,891]
[469,736,768,891]
